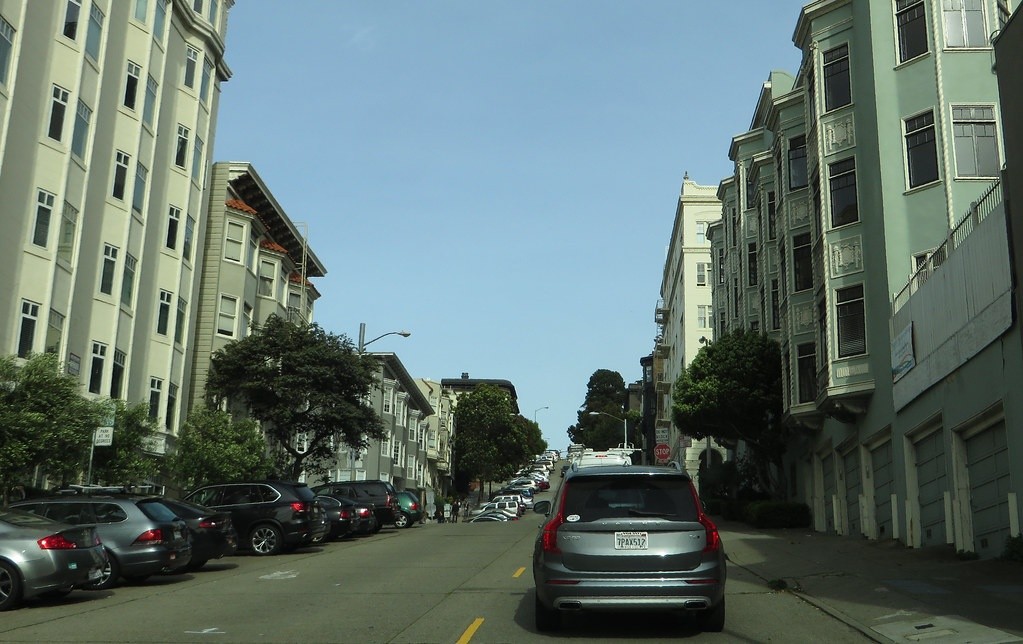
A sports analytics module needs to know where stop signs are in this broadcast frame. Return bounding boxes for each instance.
[653,444,672,462]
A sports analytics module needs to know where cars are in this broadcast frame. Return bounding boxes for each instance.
[0,505,109,612]
[394,491,424,529]
[162,495,239,573]
[463,447,635,524]
[323,493,378,541]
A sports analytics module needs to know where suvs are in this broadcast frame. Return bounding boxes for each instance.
[0,493,195,587]
[182,480,332,557]
[314,480,402,530]
[529,460,728,633]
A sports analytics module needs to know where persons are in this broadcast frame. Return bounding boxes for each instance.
[438,511,444,523]
[452,498,469,524]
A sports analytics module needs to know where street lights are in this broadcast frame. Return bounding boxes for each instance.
[349,323,411,481]
[534,407,550,426]
[588,410,628,450]
